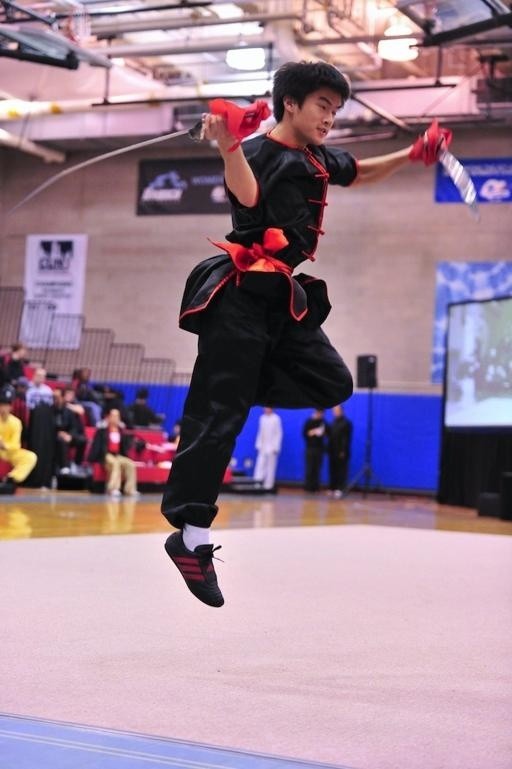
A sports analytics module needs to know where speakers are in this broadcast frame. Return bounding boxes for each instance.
[357,355,377,387]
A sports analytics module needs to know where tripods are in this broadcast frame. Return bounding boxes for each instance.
[343,388,397,501]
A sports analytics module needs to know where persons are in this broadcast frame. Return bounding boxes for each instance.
[253,408,284,494]
[0,342,183,498]
[303,406,326,493]
[327,404,353,501]
[160,62,445,607]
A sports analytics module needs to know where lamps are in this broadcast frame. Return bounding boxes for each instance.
[378,23,419,62]
[226,28,265,71]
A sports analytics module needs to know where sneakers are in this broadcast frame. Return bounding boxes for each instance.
[165,531,224,607]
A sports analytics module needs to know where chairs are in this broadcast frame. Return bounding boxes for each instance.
[0,348,233,492]
